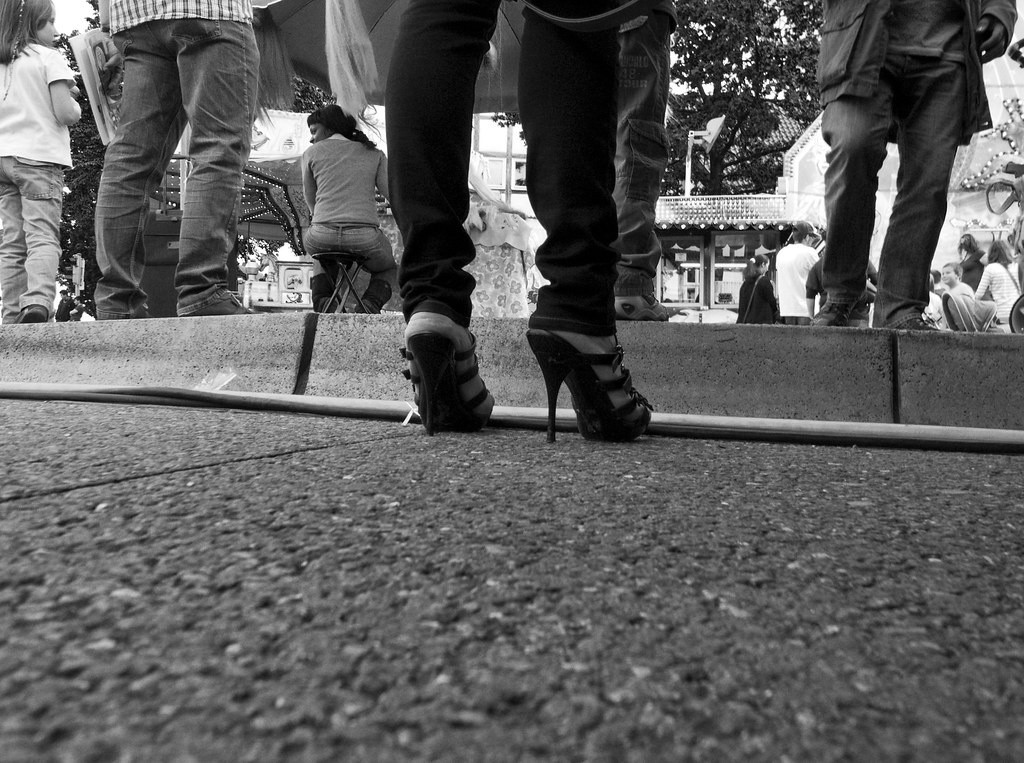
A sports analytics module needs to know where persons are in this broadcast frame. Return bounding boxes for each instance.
[95,0,260,318]
[776,220,820,325]
[941,263,975,300]
[810,0,1019,329]
[931,270,946,297]
[70,296,83,321]
[806,256,878,327]
[958,234,1024,334]
[0,0,82,323]
[302,103,398,313]
[736,254,781,324]
[922,274,950,330]
[55,290,76,322]
[384,0,679,445]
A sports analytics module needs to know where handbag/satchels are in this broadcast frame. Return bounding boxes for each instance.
[743,275,773,325]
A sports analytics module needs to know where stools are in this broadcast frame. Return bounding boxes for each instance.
[312,251,371,314]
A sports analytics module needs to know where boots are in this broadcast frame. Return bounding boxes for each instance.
[356,279,393,315]
[310,274,344,315]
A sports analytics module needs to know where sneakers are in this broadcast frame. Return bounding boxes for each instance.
[613,293,671,322]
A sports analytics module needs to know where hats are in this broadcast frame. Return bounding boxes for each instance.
[793,221,820,242]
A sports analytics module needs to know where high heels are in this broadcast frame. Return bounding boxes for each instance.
[525,324,655,444]
[400,302,496,436]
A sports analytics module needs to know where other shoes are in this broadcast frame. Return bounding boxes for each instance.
[134,303,153,318]
[191,292,263,314]
[811,298,870,326]
[13,304,47,322]
[895,314,950,331]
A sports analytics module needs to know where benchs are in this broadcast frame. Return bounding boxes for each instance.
[253,302,312,312]
